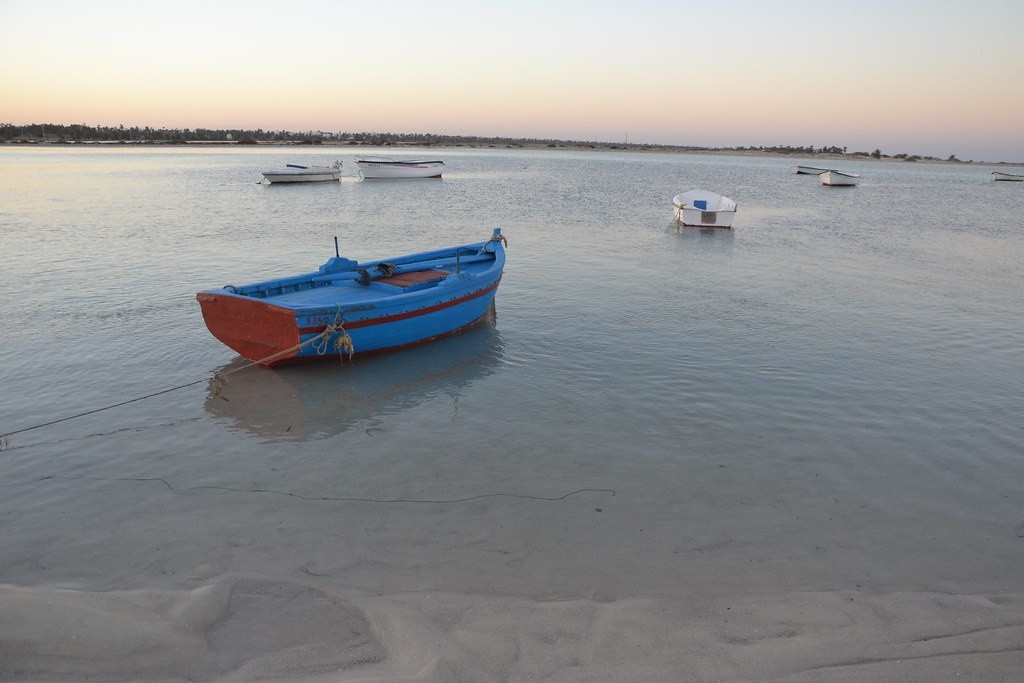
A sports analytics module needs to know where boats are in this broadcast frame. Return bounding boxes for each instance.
[354,154,446,179]
[990,171,1024,181]
[674,187,740,231]
[197,227,509,370]
[0,137,567,149]
[818,168,862,187]
[797,165,829,174]
[589,143,650,152]
[262,161,342,182]
